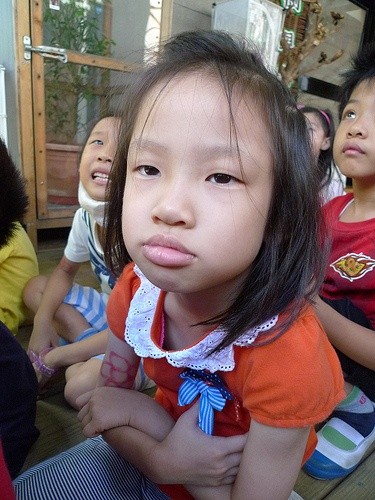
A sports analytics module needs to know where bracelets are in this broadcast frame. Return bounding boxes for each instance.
[30,347,55,377]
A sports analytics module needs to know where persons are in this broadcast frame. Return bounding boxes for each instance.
[1,29,375,500]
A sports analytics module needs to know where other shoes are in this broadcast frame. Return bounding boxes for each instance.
[302,401,375,480]
